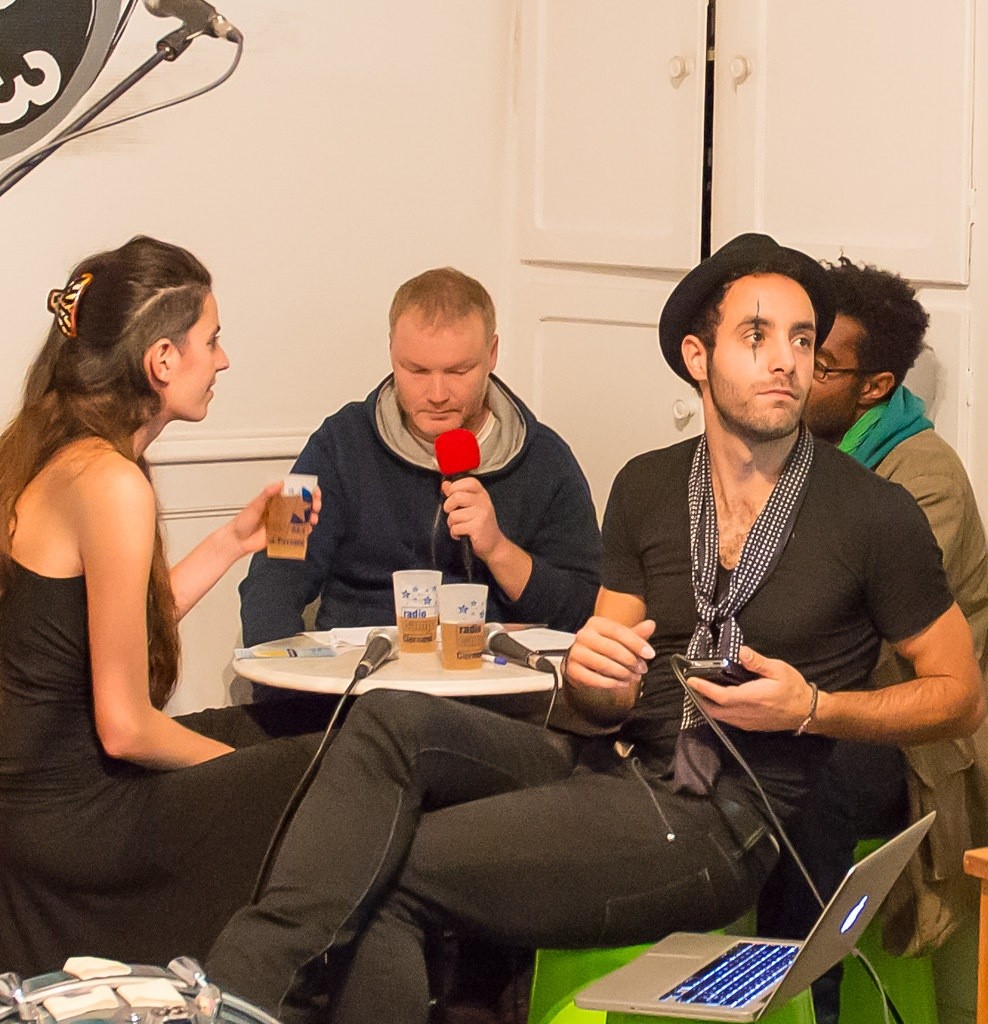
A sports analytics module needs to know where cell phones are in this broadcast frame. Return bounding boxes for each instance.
[681,656,760,689]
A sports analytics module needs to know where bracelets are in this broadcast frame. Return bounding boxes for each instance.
[792,682,817,737]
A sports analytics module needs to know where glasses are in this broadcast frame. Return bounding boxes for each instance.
[813,358,896,384]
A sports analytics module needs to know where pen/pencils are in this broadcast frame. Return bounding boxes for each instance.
[480,652,507,666]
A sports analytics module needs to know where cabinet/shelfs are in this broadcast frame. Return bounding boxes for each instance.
[512,0,976,533]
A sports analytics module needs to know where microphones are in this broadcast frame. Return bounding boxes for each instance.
[144,0,243,43]
[355,626,401,679]
[483,621,555,673]
[432,428,480,573]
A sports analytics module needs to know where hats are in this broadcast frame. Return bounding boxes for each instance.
[659,233,837,389]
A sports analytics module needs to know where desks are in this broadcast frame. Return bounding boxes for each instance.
[234,624,578,699]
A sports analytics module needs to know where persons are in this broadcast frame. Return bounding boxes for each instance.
[1,236,347,966]
[443,262,988,1024]
[204,232,988,1024]
[240,269,603,1007]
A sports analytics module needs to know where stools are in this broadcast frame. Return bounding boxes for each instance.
[527,836,940,1024]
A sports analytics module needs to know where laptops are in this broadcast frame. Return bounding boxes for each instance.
[574,810,938,1022]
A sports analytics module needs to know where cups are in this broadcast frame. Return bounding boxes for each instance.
[267,470,319,561]
[391,570,443,653]
[436,583,488,670]
